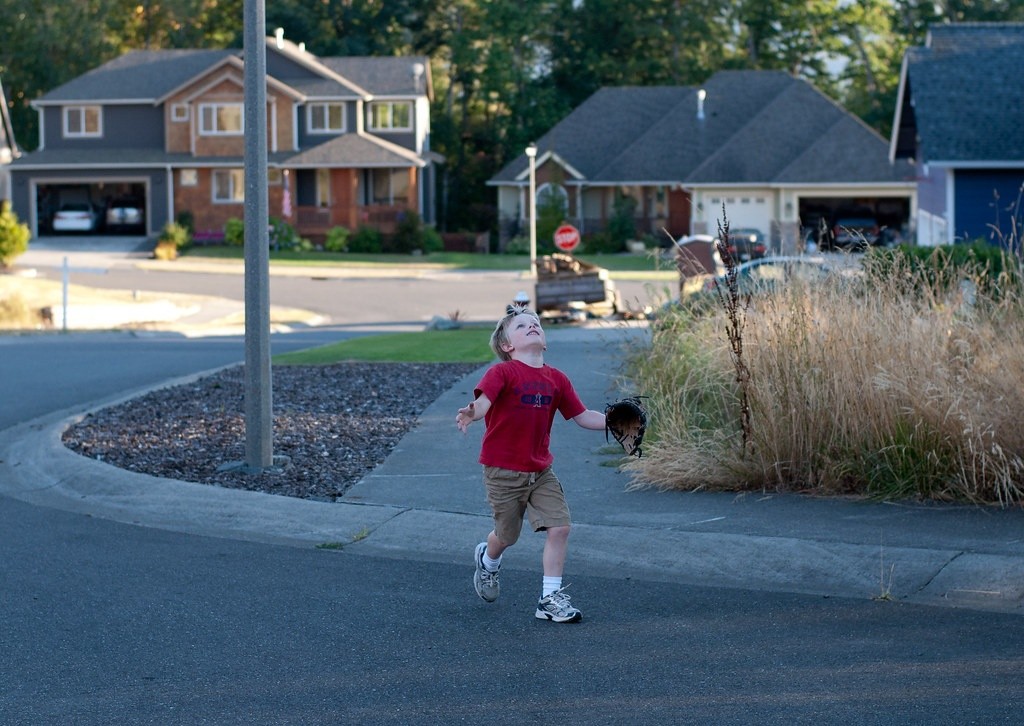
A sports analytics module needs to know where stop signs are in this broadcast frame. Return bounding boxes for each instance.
[553,225,581,253]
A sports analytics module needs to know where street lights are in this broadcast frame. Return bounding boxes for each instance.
[525,141,539,317]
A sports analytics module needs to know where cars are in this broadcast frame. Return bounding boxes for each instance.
[105,197,144,230]
[716,226,774,272]
[831,208,881,248]
[53,202,96,236]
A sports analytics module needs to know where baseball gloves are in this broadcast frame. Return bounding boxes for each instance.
[603,394,650,460]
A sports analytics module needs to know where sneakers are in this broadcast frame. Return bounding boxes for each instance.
[535,583,583,622]
[474,542,501,604]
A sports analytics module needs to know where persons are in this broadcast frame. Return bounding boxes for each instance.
[455,304,610,622]
